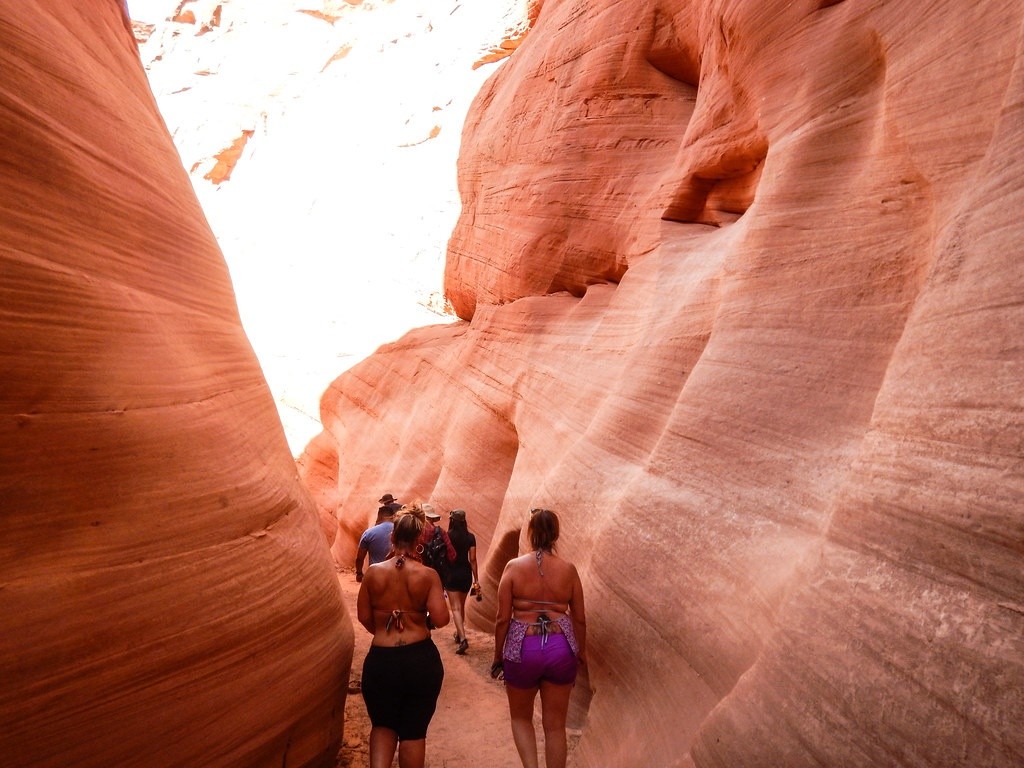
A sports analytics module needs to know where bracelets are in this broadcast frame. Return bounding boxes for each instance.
[356,572,362,575]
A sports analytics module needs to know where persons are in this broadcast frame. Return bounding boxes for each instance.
[379,494,406,512]
[355,506,395,584]
[419,504,456,571]
[446,509,481,654]
[357,503,450,768]
[488,508,588,768]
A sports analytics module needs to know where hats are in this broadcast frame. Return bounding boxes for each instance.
[448,510,466,521]
[419,504,441,522]
[378,494,398,504]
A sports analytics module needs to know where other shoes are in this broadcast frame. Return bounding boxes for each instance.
[454,632,460,643]
[456,639,468,654]
